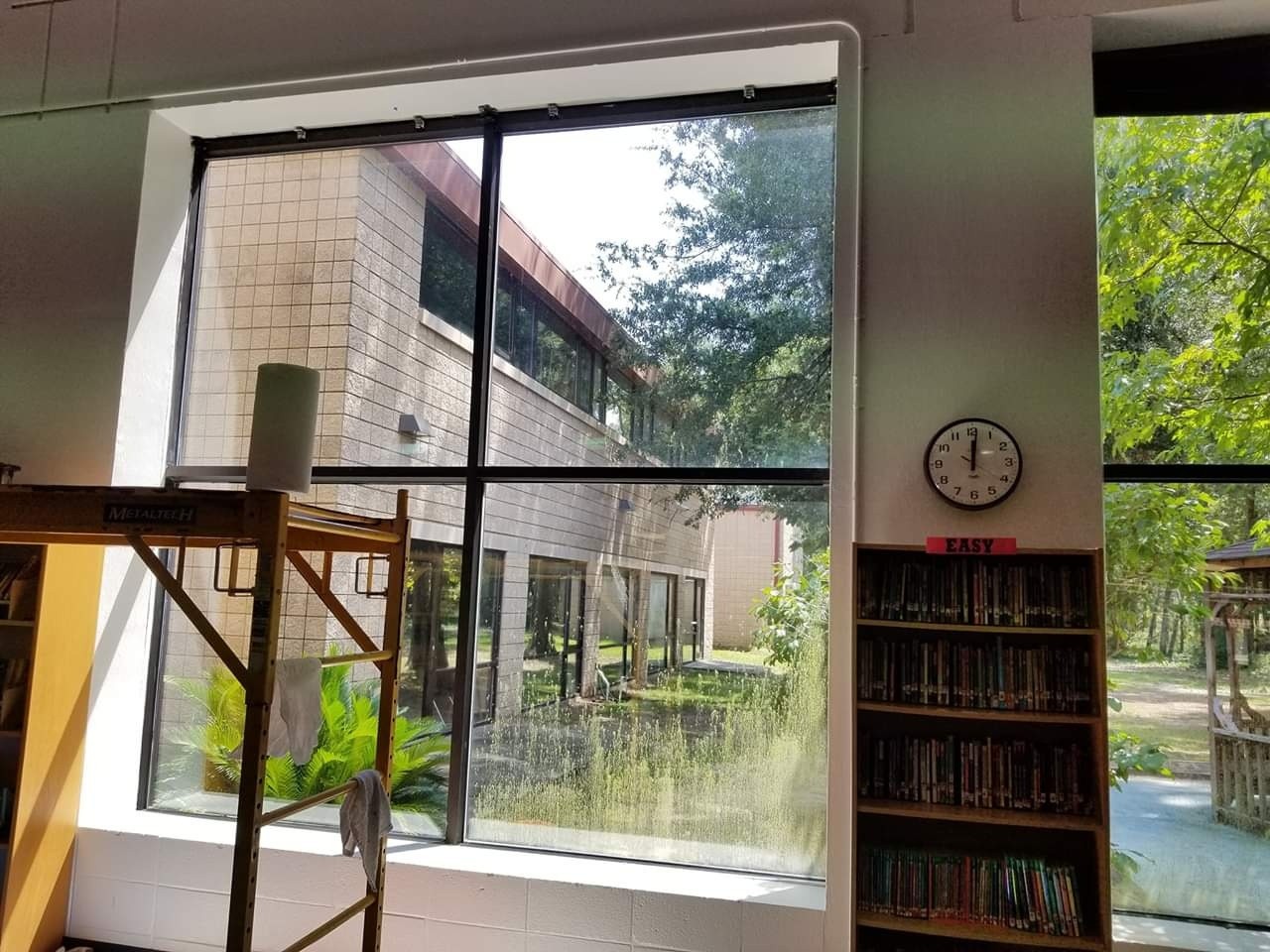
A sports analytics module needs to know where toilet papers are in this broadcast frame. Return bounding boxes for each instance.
[246,361,323,494]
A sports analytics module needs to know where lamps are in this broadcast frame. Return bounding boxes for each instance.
[398,413,439,440]
[618,499,637,512]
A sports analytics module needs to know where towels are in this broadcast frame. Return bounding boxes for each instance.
[265,656,327,766]
[338,768,395,893]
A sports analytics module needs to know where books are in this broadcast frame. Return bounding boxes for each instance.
[857,834,1100,938]
[856,632,1101,718]
[0,555,41,842]
[858,721,1102,820]
[856,552,1101,629]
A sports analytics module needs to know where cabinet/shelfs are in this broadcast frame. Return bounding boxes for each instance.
[0,476,412,952]
[850,541,1112,952]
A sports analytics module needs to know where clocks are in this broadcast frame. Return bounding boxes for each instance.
[920,417,1026,512]
